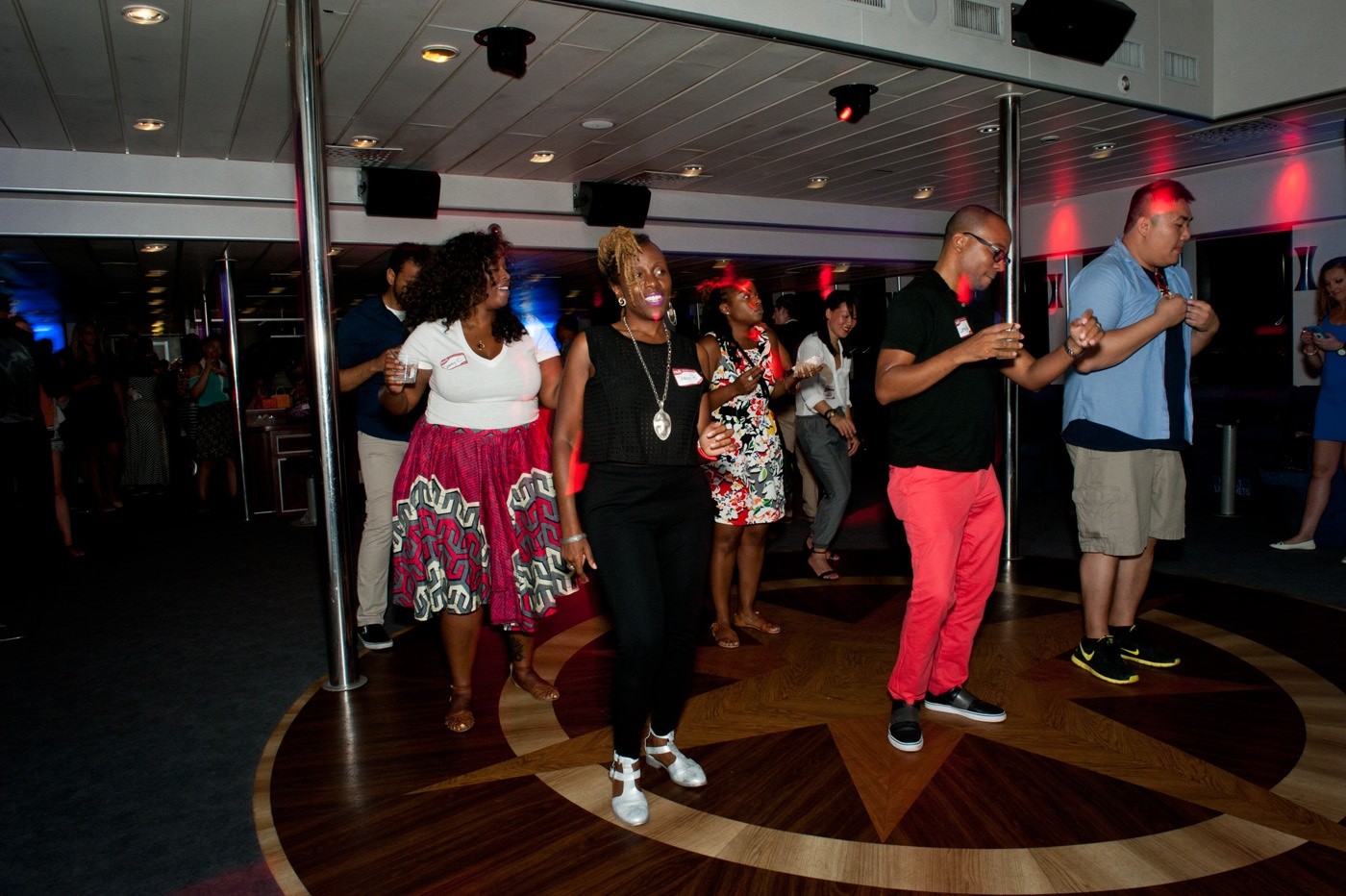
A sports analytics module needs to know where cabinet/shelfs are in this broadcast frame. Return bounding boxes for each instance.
[244,424,318,516]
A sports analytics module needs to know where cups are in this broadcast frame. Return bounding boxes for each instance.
[997,326,1019,359]
[390,350,419,383]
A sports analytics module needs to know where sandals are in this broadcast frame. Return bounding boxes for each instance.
[710,623,740,648]
[510,664,560,701]
[445,685,474,732]
[733,611,781,634]
[807,551,841,580]
[806,535,841,560]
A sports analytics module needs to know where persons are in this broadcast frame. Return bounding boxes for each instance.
[0,223,861,827]
[873,204,1106,751]
[1268,257,1346,564]
[1061,179,1219,685]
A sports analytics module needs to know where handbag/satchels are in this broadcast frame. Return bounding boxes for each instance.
[215,419,237,439]
[783,450,807,511]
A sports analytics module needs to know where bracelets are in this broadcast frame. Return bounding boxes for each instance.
[697,439,721,461]
[825,409,839,420]
[1303,346,1318,355]
[561,533,586,544]
[1063,340,1087,359]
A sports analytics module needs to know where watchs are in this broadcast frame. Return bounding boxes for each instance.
[1337,342,1346,355]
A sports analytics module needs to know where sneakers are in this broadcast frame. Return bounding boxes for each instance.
[925,685,1007,722]
[1114,625,1181,667]
[1071,635,1139,684]
[887,699,924,752]
[357,623,393,649]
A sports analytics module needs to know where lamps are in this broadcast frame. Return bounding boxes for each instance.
[829,83,878,124]
[1294,246,1318,291]
[1048,274,1063,308]
[474,27,536,80]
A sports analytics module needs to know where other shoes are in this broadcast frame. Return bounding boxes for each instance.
[802,508,815,522]
[98,504,116,511]
[113,499,124,508]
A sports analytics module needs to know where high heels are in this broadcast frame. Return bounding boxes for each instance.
[609,750,649,826]
[643,722,707,787]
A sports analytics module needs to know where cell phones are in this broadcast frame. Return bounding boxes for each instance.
[1311,329,1325,339]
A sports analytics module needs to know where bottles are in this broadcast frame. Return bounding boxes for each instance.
[256,379,265,410]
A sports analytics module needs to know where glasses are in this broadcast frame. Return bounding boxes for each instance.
[963,232,1010,269]
[1155,268,1171,297]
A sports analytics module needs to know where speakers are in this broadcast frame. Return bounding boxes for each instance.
[572,181,651,228]
[1011,0,1137,66]
[358,168,441,219]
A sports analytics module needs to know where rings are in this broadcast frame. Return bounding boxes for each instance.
[567,564,576,570]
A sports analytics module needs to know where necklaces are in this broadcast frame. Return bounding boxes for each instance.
[622,315,672,441]
[463,317,493,350]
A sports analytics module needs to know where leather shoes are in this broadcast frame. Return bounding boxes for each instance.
[1269,539,1316,550]
[1342,557,1346,563]
